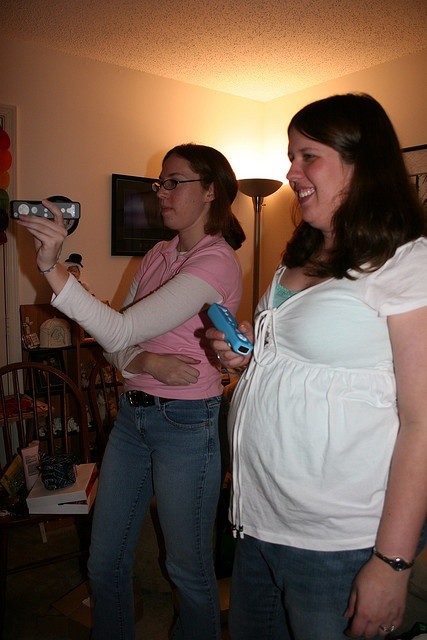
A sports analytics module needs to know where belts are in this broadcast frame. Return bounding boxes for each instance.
[125,389,175,406]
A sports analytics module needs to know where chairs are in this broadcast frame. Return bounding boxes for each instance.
[0,361,89,582]
[87,360,124,459]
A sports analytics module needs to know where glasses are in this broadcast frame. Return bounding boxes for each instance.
[152,179,205,190]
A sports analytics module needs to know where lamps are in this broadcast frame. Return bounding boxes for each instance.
[237,180,282,328]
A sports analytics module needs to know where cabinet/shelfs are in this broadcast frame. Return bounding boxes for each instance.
[18,304,123,450]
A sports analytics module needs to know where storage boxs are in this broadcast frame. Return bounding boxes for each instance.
[26,462,99,514]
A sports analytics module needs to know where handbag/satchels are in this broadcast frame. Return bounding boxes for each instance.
[38,449,80,491]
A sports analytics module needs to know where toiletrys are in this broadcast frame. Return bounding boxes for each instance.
[21,444,40,491]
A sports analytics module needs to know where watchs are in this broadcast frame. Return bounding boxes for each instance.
[373,546,413,572]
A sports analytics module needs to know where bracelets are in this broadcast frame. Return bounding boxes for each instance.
[40,260,58,274]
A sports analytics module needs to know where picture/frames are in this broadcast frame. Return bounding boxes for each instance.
[401,144,427,212]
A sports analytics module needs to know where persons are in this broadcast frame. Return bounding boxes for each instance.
[15,142,246,640]
[204,91,426,640]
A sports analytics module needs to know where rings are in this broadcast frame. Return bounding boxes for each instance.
[381,624,395,631]
